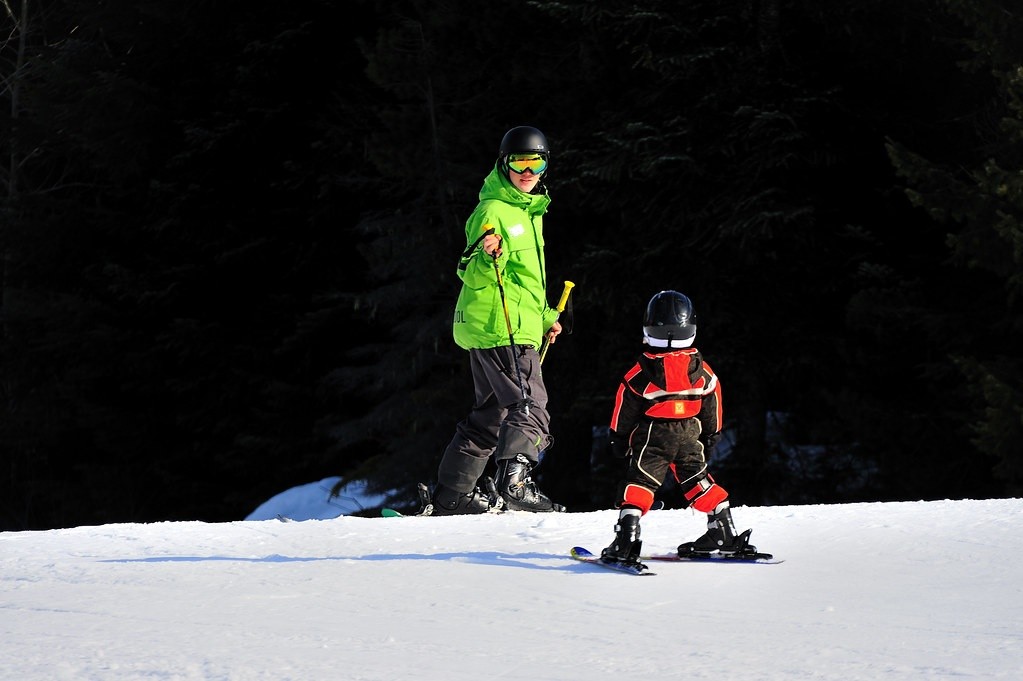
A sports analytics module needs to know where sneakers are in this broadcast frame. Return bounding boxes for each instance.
[492,453,553,511]
[431,483,490,515]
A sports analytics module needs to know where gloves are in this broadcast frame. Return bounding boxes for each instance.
[698,433,721,461]
[608,430,629,458]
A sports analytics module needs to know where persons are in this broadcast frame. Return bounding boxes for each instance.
[429,125,562,513]
[603,291,741,563]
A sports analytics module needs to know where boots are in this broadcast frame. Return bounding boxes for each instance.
[693,501,737,551]
[601,509,642,561]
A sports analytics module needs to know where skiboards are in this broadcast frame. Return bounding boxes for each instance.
[380,507,405,517]
[569,546,787,576]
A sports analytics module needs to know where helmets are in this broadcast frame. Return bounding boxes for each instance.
[644,291,696,339]
[498,125,551,168]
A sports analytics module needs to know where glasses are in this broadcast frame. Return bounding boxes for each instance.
[507,152,548,175]
